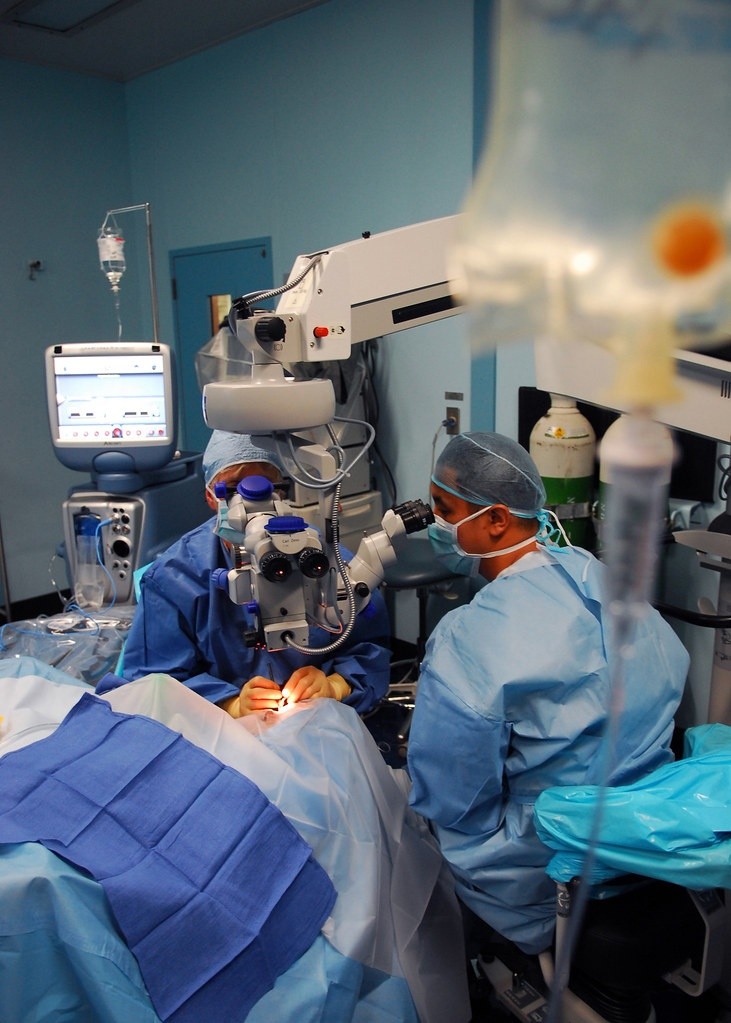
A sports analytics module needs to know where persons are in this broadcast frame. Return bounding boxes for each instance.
[123,426,395,715]
[234,698,322,732]
[407,431,691,956]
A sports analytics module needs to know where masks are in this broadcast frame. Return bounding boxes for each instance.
[207,486,246,546]
[427,505,494,577]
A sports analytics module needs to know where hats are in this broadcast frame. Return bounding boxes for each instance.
[202,426,297,488]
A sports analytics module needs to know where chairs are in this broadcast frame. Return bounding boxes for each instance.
[478,722,731,1023]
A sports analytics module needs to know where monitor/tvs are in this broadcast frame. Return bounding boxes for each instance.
[42,340,178,474]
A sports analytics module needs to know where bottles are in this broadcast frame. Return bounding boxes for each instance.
[97,228,126,285]
